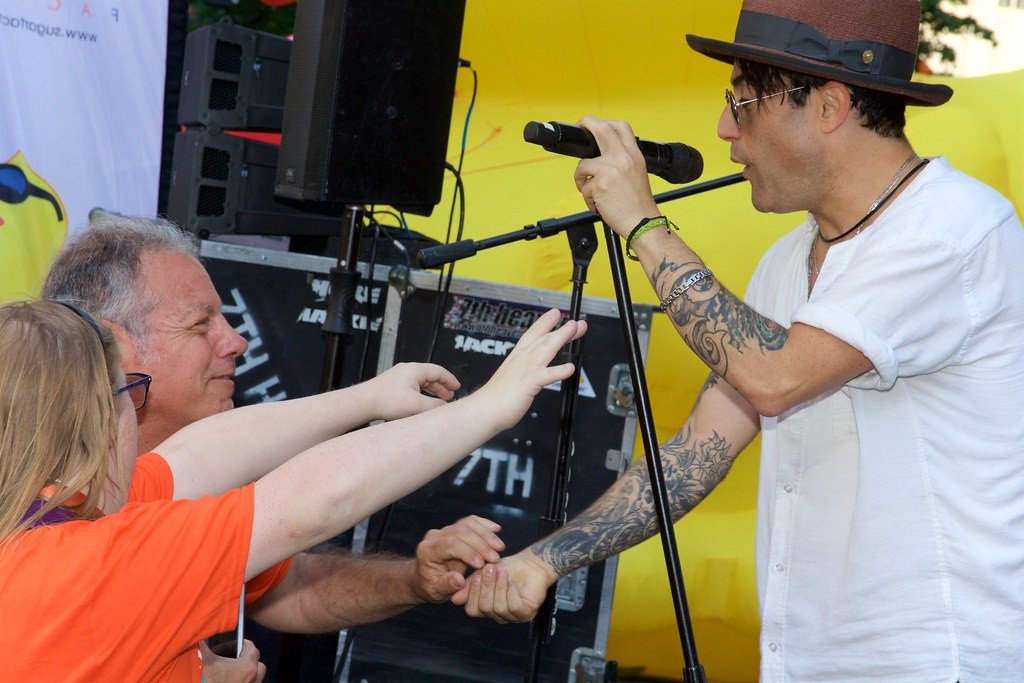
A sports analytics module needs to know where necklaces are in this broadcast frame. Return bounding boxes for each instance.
[808,154,930,283]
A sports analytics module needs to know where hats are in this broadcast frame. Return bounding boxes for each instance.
[685,2,955,106]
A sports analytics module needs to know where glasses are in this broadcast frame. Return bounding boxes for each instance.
[108,369,153,417]
[725,78,855,124]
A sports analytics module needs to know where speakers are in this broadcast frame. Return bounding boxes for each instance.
[167,0,468,253]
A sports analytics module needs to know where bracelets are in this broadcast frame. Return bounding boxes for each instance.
[660,269,713,313]
[626,216,680,261]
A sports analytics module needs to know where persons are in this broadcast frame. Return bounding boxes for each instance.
[41,217,504,683]
[452,1,1023,683]
[0,300,587,683]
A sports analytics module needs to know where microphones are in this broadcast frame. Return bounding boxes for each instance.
[523,120,704,185]
